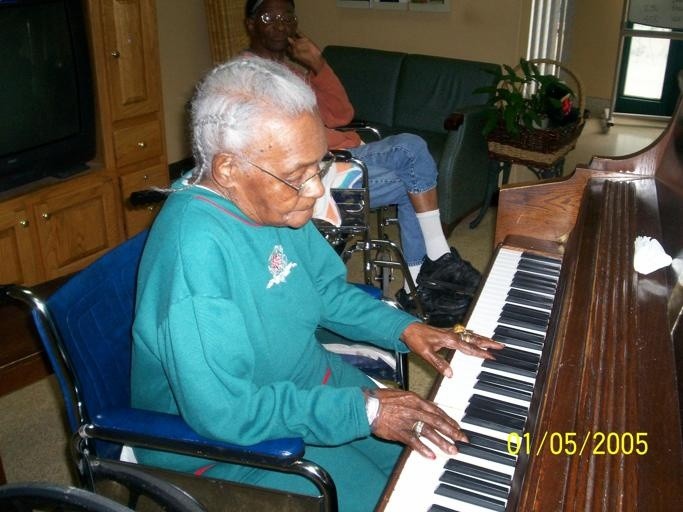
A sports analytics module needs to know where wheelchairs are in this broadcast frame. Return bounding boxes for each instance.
[312,122,440,321]
[0,184,410,511]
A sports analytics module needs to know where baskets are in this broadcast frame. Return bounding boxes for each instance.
[478,58,587,169]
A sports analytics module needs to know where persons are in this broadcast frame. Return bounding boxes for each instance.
[130,54,505,512]
[242,0,483,314]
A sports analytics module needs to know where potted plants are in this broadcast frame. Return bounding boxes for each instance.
[451,54,588,152]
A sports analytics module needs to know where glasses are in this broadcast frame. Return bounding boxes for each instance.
[256,12,299,26]
[233,149,336,192]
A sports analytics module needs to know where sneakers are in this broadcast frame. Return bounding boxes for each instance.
[395,246,484,329]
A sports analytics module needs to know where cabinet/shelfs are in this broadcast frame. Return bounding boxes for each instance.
[1,0,170,287]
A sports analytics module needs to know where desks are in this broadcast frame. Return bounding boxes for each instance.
[469,142,575,232]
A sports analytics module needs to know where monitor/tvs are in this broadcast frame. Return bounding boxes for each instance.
[0,0,96,196]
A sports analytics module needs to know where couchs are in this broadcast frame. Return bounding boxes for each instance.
[318,43,504,240]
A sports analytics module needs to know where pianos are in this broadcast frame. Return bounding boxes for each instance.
[376,100,682,512]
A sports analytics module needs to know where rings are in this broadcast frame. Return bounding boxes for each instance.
[461,333,464,341]
[412,422,417,431]
[415,421,424,433]
[454,323,465,334]
[465,332,472,343]
